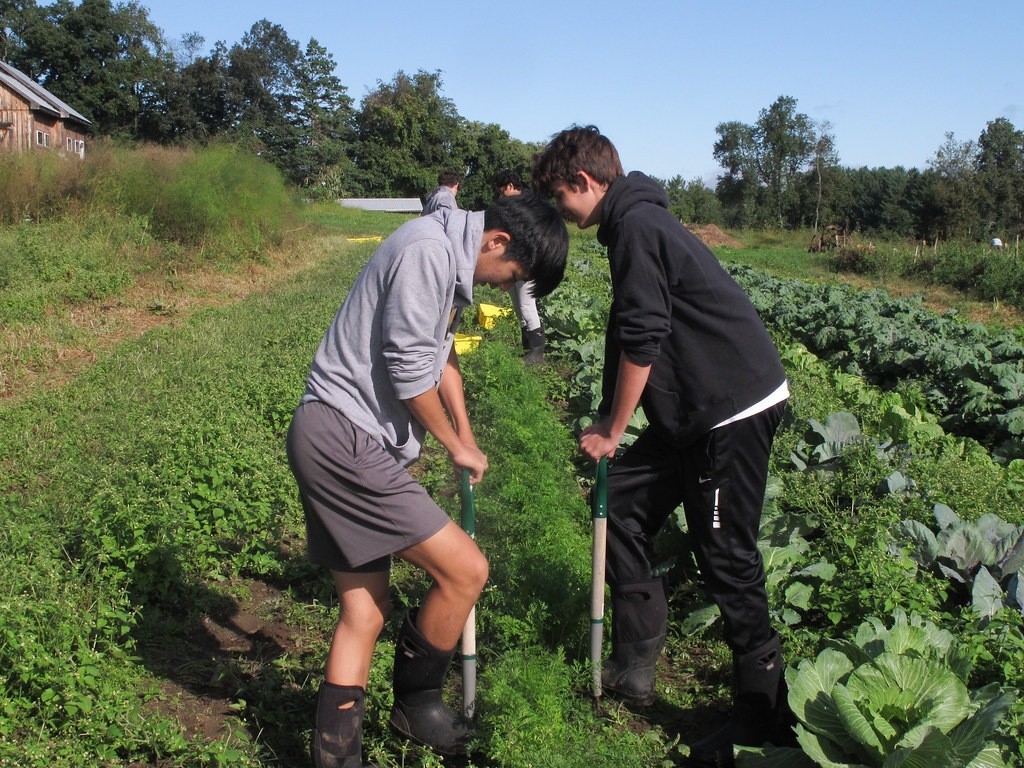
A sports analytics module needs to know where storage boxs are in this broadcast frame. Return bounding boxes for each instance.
[479,303,512,329]
[454,333,483,354]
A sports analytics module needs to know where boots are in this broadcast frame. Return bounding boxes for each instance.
[690,629,795,766]
[590,578,667,706]
[311,679,362,768]
[520,326,530,357]
[519,327,544,365]
[390,608,478,762]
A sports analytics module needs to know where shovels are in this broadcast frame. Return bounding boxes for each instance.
[447,468,483,768]
[569,455,617,727]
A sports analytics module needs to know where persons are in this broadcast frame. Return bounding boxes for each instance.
[495,170,546,366]
[989,237,1002,251]
[533,127,802,746]
[420,171,462,217]
[281,196,568,768]
